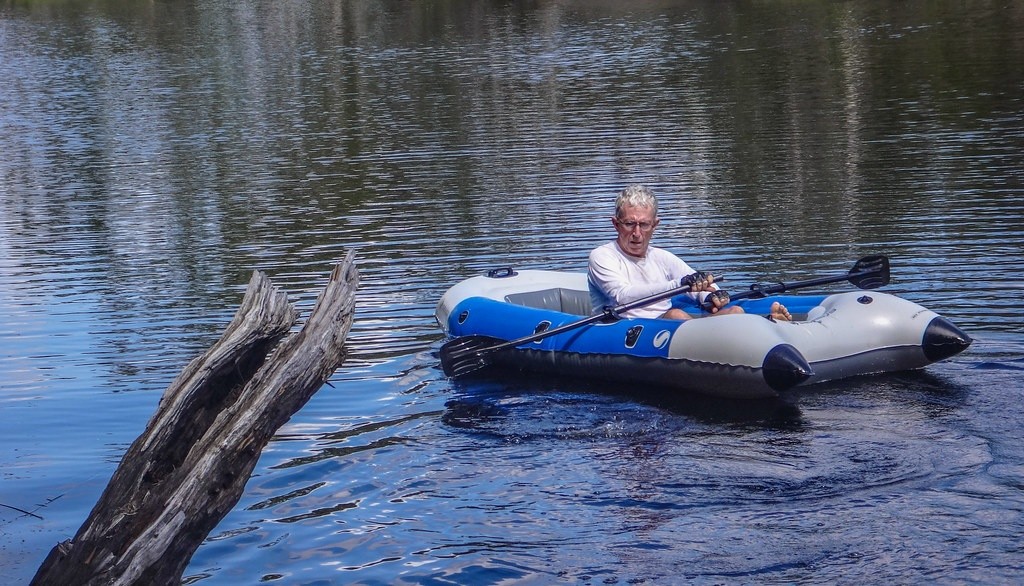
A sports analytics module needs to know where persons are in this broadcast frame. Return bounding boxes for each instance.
[587,183,794,324]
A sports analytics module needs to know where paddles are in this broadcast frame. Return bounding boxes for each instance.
[438,274,729,380]
[701,254,892,310]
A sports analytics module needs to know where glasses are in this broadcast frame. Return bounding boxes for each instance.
[616,217,655,232]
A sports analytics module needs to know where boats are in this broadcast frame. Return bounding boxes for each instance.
[433,266,973,402]
